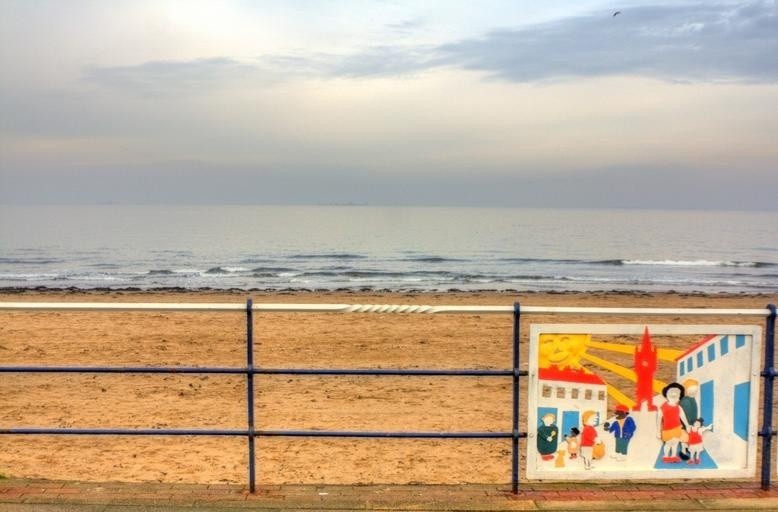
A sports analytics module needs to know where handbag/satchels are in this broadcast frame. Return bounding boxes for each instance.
[591,439,606,460]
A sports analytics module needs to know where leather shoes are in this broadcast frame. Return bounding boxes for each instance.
[662,447,700,465]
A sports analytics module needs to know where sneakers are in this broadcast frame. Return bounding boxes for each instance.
[611,453,628,462]
